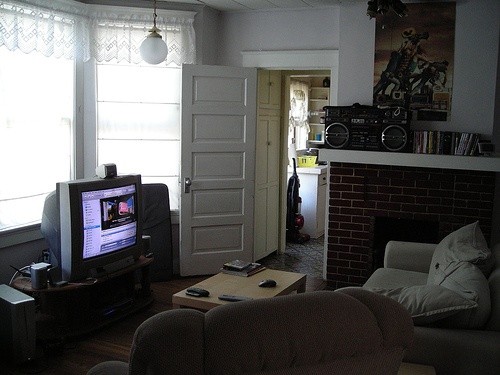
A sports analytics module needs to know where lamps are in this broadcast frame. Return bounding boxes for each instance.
[139,0,168,65]
[366,0,409,21]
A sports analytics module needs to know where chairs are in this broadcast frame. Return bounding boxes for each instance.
[85,287,415,375]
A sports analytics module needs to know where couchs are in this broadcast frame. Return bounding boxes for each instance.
[334,240,500,375]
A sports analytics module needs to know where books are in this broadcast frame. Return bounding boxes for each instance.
[411,129,479,156]
[222,260,265,277]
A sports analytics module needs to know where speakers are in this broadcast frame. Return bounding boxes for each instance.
[30,263,51,290]
[141,235,151,257]
[95,164,117,178]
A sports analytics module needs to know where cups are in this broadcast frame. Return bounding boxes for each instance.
[315,133,321,141]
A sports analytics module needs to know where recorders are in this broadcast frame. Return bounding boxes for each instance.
[323,104,414,153]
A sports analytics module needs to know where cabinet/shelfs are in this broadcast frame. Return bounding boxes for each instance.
[309,86,330,144]
[287,172,327,239]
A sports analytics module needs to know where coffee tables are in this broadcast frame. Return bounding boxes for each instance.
[172,268,307,311]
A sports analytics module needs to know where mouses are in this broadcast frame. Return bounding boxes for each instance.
[258,279,276,287]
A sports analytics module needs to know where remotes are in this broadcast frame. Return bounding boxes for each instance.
[218,295,253,302]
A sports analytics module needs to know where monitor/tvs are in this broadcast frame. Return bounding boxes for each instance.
[40,174,142,284]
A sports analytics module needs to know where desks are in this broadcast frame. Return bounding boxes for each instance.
[12,254,153,368]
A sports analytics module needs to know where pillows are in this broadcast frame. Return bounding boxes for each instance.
[429,220,495,279]
[381,285,478,326]
[434,259,492,330]
